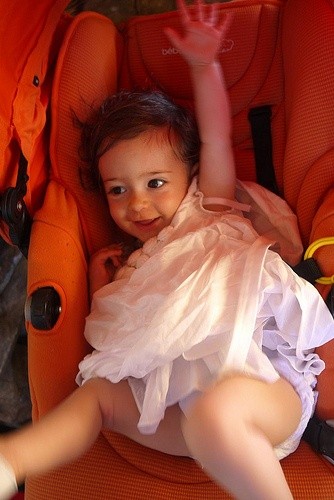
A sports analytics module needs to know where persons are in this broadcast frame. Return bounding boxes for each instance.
[1,1,325,500]
